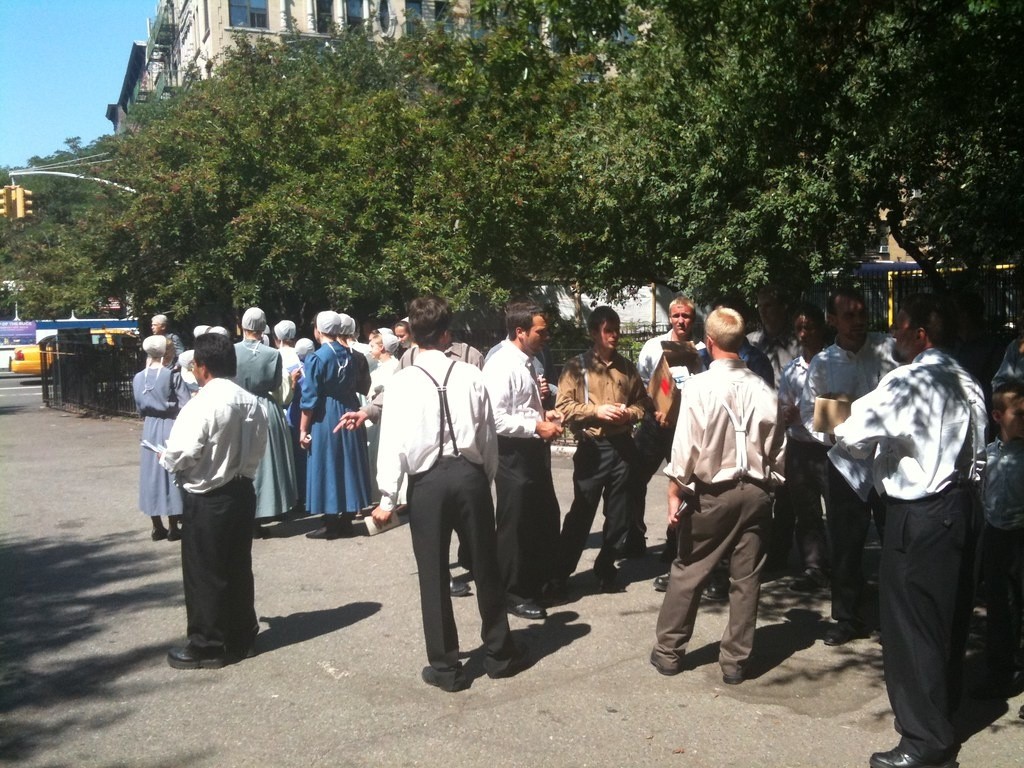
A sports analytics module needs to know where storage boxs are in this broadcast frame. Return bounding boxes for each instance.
[813,391,862,435]
[647,340,707,424]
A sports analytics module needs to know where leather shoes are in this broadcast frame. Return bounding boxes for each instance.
[450,578,471,597]
[512,601,548,619]
[167,640,255,669]
[824,625,849,646]
[869,747,925,768]
[654,575,669,592]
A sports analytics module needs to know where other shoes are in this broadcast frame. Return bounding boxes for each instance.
[488,642,530,679]
[307,525,356,540]
[152,526,182,541]
[786,574,819,591]
[422,666,439,687]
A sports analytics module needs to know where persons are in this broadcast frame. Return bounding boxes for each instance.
[158,333,269,669]
[132,306,372,541]
[977,307,1024,717]
[834,292,990,768]
[333,294,910,646]
[371,293,529,692]
[651,305,787,685]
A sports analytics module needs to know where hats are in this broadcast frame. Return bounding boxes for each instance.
[316,311,356,336]
[242,307,266,331]
[400,316,409,324]
[142,335,166,394]
[193,325,227,338]
[377,327,401,352]
[273,320,296,341]
[295,338,315,360]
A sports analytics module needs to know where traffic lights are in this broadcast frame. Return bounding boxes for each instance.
[16,187,33,220]
[0,187,10,219]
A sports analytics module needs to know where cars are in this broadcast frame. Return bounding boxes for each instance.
[9,327,141,375]
